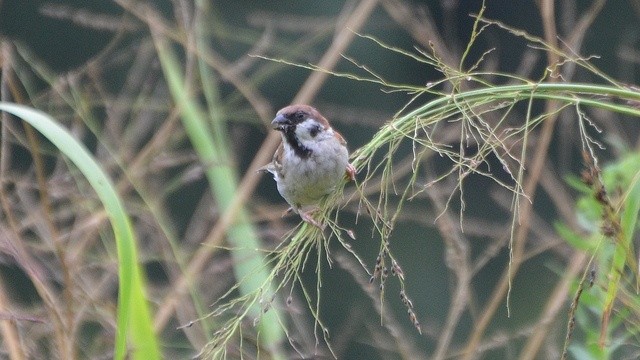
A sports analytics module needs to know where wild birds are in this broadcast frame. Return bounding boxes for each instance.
[255,104,357,232]
